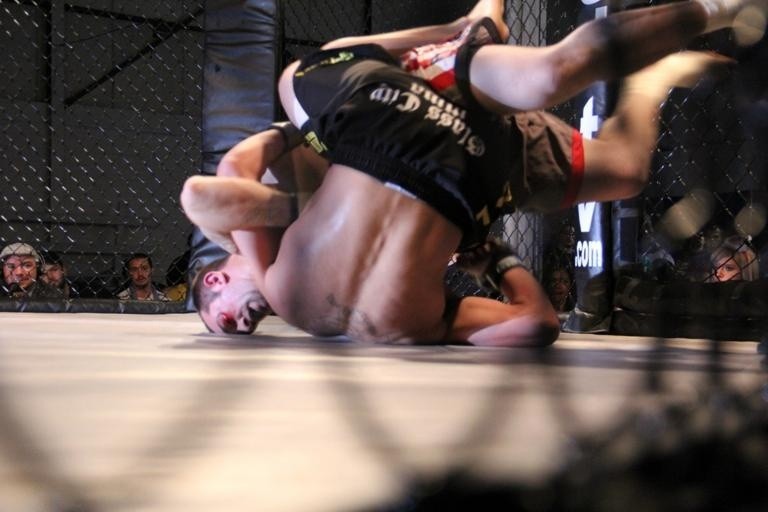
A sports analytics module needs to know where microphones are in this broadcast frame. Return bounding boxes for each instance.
[0,282,18,294]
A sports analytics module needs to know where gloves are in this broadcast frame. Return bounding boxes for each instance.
[269,120,308,154]
[447,236,531,295]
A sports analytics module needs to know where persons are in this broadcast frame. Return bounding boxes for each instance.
[180,0,768,335]
[214,0,738,349]
[38,253,81,298]
[0,243,59,301]
[116,253,169,301]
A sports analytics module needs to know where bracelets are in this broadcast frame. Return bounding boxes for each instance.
[287,190,300,226]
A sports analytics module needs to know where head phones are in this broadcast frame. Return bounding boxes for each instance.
[0,248,46,279]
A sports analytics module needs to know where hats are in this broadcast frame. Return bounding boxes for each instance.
[0,242,39,259]
